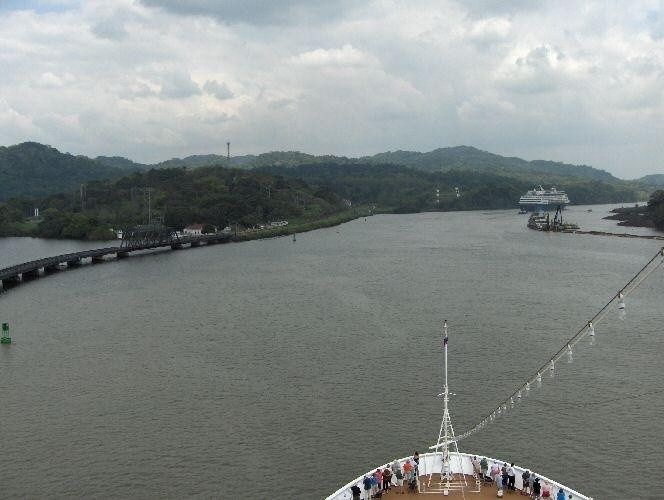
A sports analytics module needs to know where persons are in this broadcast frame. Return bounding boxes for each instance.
[472,456,573,500]
[351,451,419,500]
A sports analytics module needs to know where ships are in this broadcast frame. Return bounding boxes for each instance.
[517,184,571,213]
